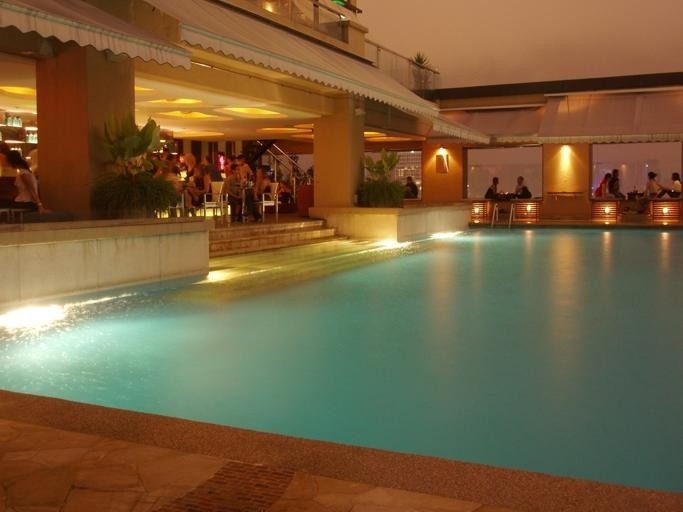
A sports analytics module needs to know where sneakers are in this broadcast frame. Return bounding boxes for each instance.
[230,215,262,225]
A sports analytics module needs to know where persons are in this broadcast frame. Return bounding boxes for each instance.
[485,177,502,198]
[0,141,52,214]
[595,173,616,199]
[655,173,682,198]
[512,176,532,199]
[637,172,658,212]
[611,169,627,215]
[148,151,294,223]
[401,176,418,199]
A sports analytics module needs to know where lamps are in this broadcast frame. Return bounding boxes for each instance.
[437,144,448,150]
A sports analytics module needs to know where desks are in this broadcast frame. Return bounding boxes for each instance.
[620,199,646,215]
[496,196,516,214]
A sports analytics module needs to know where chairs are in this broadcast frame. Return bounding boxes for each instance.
[156,175,281,221]
